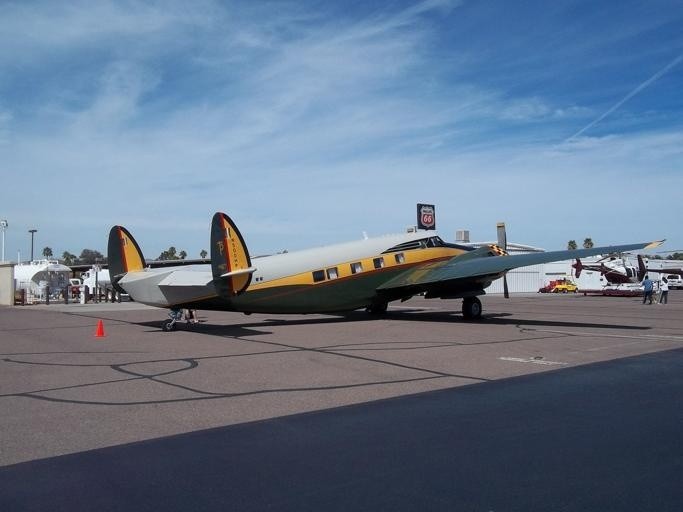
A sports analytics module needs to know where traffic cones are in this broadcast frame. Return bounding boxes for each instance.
[95,320,107,337]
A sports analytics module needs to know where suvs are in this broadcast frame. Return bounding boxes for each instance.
[666,274,683,290]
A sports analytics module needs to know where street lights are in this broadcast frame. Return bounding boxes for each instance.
[28,230,37,262]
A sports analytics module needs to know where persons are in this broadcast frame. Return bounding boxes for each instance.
[186,309,199,323]
[658,277,668,304]
[640,275,653,305]
